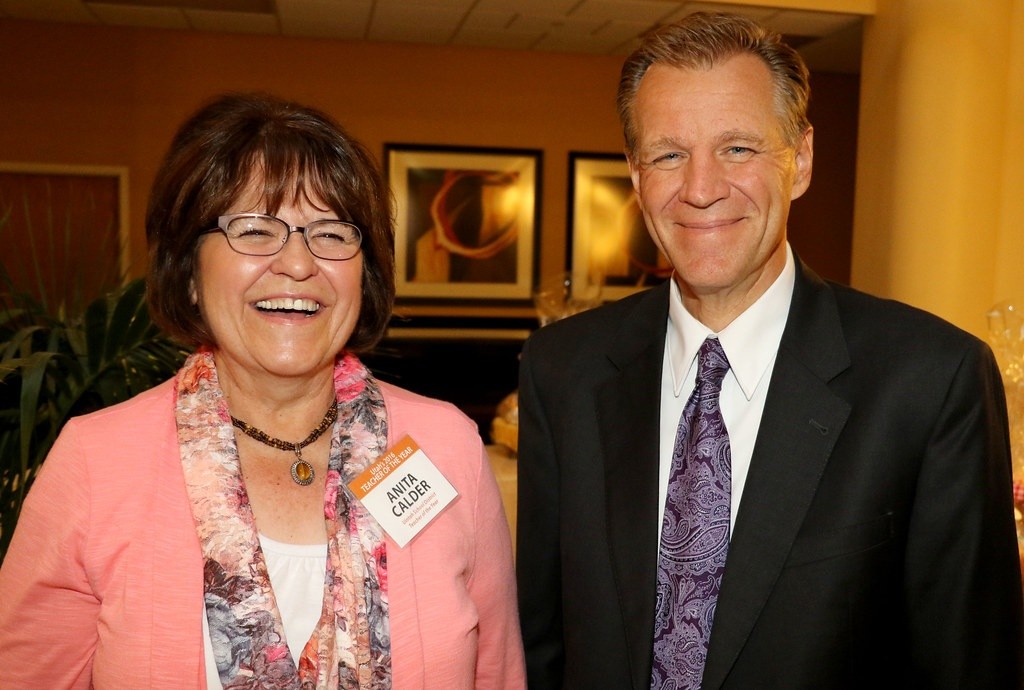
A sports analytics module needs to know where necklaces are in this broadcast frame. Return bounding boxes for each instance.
[230,397,339,486]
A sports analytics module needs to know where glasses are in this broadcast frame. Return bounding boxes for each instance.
[196,213,364,261]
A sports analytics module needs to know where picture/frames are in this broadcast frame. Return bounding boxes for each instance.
[382,143,540,307]
[566,150,672,306]
[0,162,131,316]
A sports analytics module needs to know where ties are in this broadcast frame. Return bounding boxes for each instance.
[647,338,734,690]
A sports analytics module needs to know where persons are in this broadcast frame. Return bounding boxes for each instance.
[515,12,1023,690]
[0,97,531,690]
